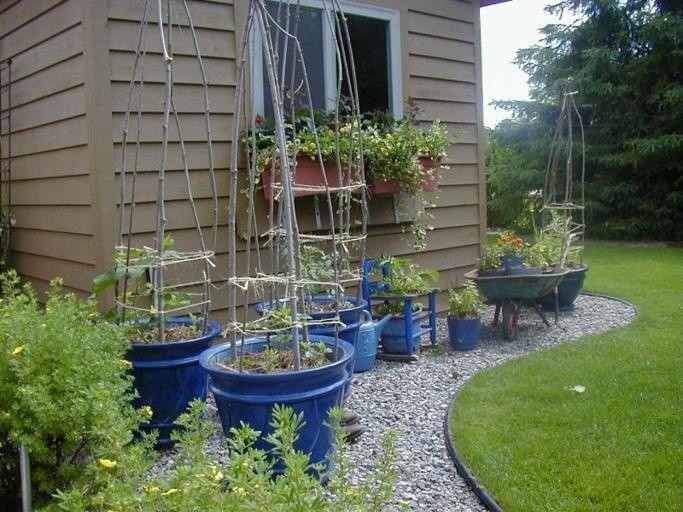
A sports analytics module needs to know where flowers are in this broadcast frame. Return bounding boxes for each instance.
[241,95,465,251]
